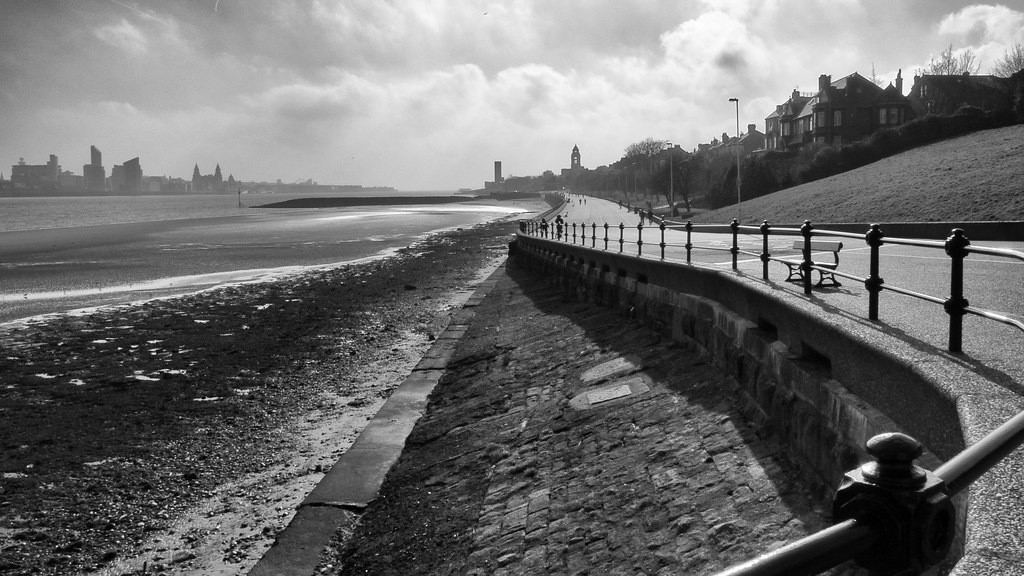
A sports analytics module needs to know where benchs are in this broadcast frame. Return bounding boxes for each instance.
[782,240,843,288]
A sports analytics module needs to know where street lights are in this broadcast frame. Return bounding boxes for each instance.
[666,142,675,221]
[631,163,638,200]
[728,98,742,226]
[624,166,630,198]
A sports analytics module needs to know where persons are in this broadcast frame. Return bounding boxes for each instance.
[618,200,632,213]
[539,214,565,240]
[638,208,654,226]
